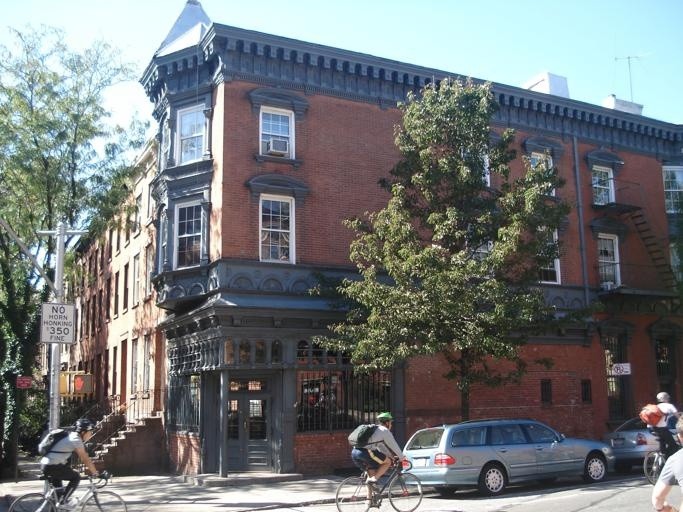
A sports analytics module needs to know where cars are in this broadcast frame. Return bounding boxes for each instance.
[245,400,327,432]
[599,412,682,474]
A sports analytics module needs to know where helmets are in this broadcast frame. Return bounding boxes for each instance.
[656,391,673,402]
[375,411,396,423]
[75,418,97,433]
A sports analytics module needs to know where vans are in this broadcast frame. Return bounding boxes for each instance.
[400,417,615,497]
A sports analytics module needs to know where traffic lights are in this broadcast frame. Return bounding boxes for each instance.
[70,373,93,394]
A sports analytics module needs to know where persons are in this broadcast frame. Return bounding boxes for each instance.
[651,415,683,512]
[40,418,99,511]
[351,413,405,507]
[647,392,680,459]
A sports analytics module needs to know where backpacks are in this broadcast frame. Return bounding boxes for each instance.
[347,423,385,449]
[638,402,673,427]
[37,426,84,457]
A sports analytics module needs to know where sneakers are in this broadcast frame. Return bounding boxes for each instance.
[55,501,77,511]
[365,478,379,491]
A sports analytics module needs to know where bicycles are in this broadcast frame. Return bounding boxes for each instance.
[643,430,683,486]
[335,455,423,512]
[7,469,127,511]
